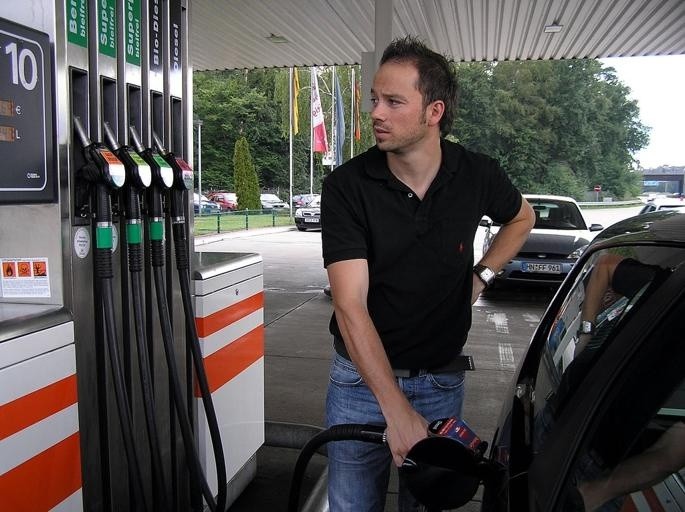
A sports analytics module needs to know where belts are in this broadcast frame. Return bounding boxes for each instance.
[331,337,476,378]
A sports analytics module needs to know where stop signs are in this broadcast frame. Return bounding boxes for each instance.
[594,185,601,193]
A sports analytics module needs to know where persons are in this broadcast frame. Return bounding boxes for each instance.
[320,36,537,512]
[535,253,685,512]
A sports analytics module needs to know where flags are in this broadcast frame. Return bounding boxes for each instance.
[290,68,302,138]
[311,67,328,159]
[333,65,345,171]
[350,64,363,144]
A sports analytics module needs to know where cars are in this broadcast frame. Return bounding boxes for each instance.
[194,192,236,213]
[259,193,288,215]
[291,193,323,231]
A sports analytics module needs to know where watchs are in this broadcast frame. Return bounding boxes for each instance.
[576,319,596,336]
[474,259,497,289]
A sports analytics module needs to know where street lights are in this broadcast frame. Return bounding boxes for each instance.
[195,120,203,216]
